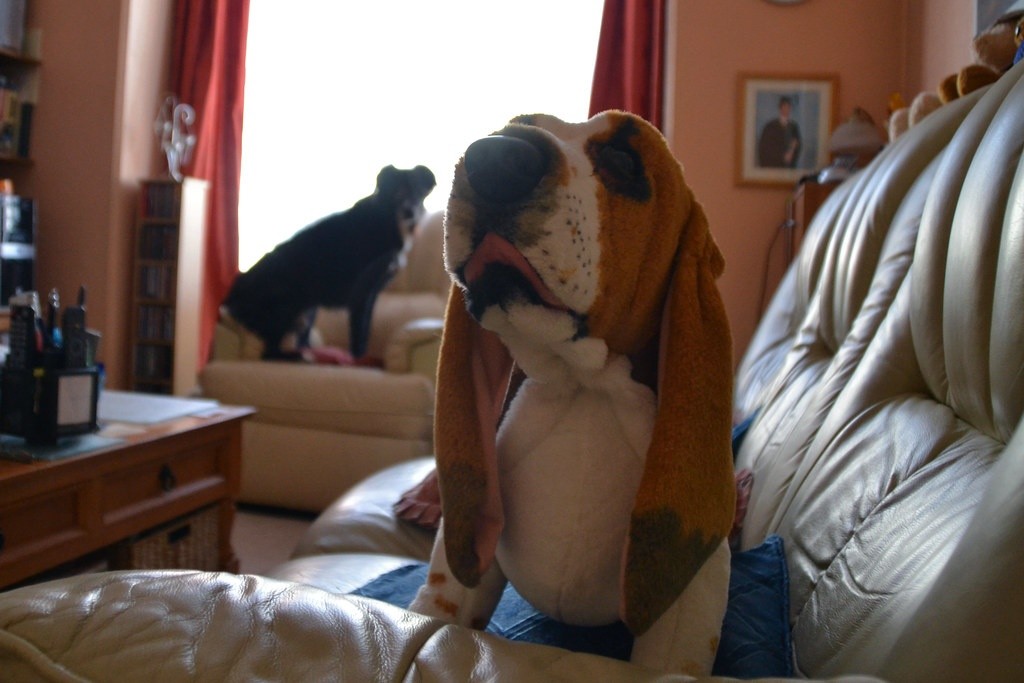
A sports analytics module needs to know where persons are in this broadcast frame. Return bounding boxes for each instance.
[758,96,802,168]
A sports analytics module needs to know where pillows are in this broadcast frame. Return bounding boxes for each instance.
[351,536,800,680]
[392,466,755,541]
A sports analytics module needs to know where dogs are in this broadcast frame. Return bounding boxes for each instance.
[217,163,438,365]
[404,107,742,677]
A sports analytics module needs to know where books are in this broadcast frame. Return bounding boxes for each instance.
[138,304,171,339]
[0,88,34,158]
[136,344,170,379]
[139,267,172,300]
[143,182,179,219]
[137,383,172,395]
[141,226,178,261]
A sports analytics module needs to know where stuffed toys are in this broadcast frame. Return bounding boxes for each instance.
[1013,17,1024,65]
[410,110,737,677]
[938,0,1024,104]
[889,93,944,143]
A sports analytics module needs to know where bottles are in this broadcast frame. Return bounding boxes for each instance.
[0,285,102,447]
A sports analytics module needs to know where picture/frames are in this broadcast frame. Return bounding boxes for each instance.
[735,70,839,190]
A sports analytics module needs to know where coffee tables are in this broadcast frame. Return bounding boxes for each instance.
[0,392,258,597]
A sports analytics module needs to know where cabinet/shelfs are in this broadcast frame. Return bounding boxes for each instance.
[130,174,211,393]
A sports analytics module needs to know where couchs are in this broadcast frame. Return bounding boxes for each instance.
[203,209,453,509]
[0,59,1024,683]
